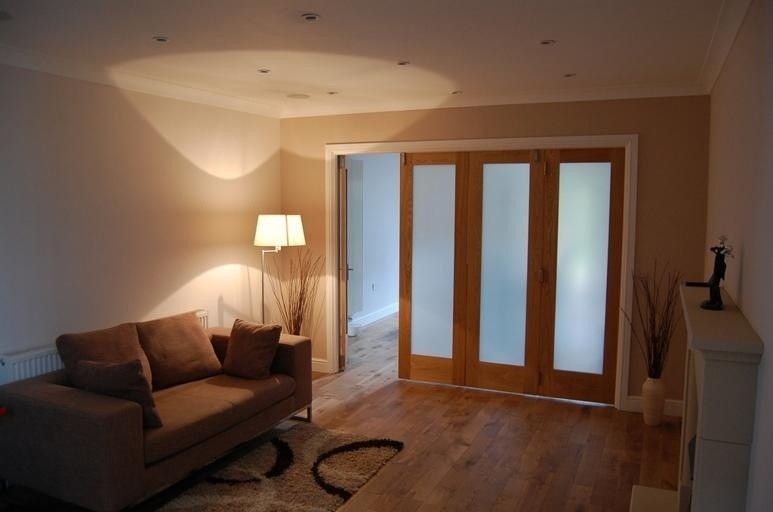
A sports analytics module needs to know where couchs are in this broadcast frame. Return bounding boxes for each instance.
[0,325,313,512]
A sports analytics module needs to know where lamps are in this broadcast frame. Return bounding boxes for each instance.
[252,214,306,325]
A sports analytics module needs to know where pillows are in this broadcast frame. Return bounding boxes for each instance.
[56,323,153,392]
[74,358,164,428]
[136,311,222,391]
[222,318,283,379]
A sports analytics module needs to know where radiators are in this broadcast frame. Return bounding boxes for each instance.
[2,311,208,385]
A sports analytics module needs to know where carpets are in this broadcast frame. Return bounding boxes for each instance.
[156,422,404,512]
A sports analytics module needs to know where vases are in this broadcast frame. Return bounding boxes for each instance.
[643,377,666,426]
